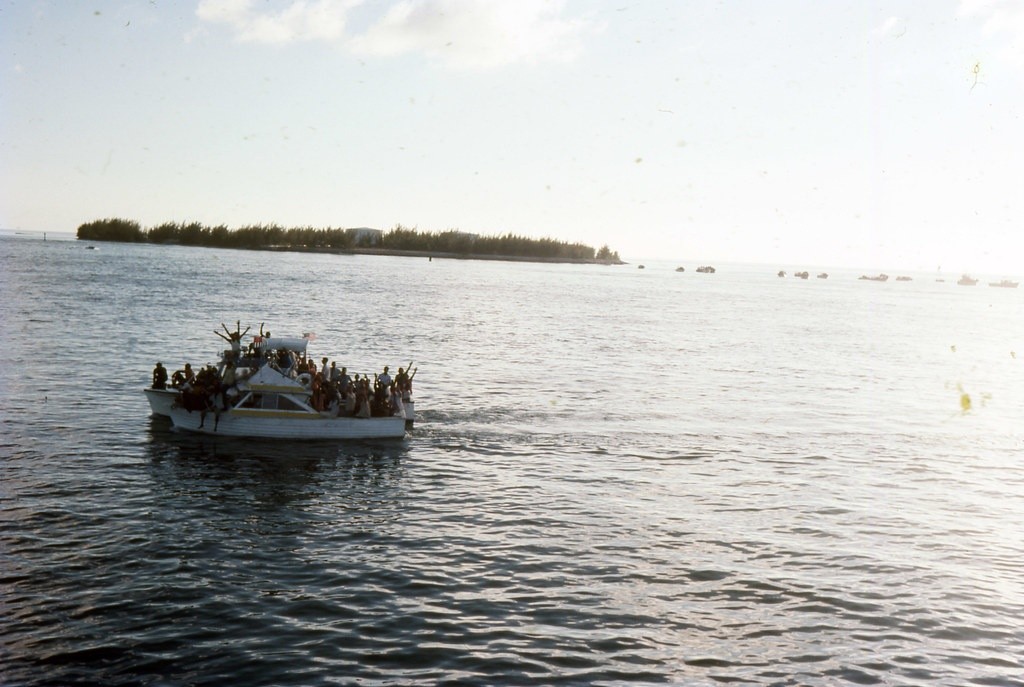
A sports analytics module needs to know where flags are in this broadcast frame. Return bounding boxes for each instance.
[302,332,316,341]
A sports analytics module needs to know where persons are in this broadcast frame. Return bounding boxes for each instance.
[152,320,417,433]
